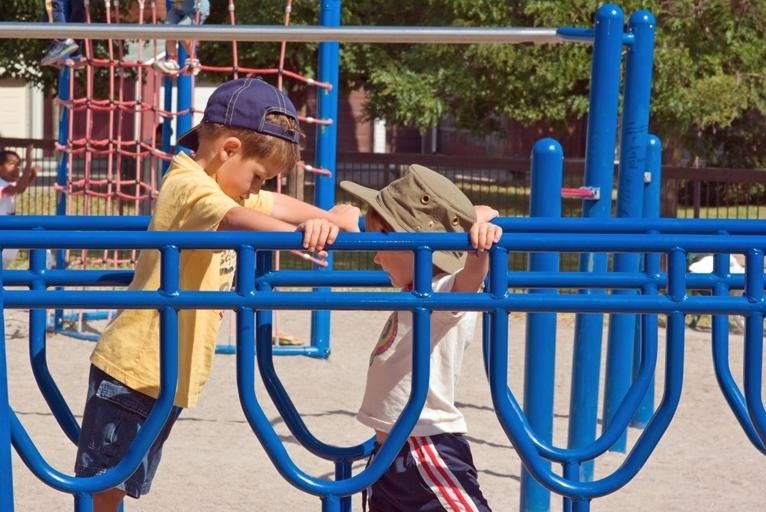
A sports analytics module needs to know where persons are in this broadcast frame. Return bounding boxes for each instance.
[1,141,41,269]
[38,1,87,67]
[75,79,361,512]
[340,163,504,512]
[151,0,211,76]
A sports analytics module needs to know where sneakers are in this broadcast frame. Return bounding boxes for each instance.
[151,60,179,77]
[181,58,201,77]
[39,38,79,66]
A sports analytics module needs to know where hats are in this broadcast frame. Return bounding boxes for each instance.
[338,163,477,274]
[176,76,300,152]
